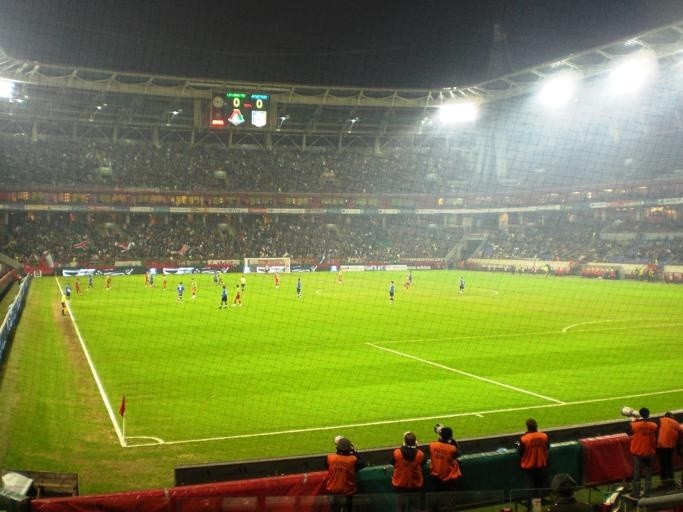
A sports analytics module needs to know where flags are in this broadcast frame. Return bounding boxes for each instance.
[117,397,126,418]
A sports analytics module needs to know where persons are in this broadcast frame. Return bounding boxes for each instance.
[59,290,67,316]
[64,281,71,301]
[324,436,368,511]
[74,278,81,295]
[0,95,683,282]
[161,274,168,290]
[425,426,464,510]
[175,282,184,304]
[217,285,228,310]
[240,275,246,291]
[147,273,156,289]
[271,274,280,289]
[545,473,600,512]
[387,280,395,305]
[190,278,196,299]
[142,270,149,288]
[390,432,428,510]
[458,275,465,294]
[655,410,682,490]
[623,407,659,498]
[230,284,241,307]
[84,274,94,292]
[105,275,111,290]
[335,270,343,285]
[213,274,225,287]
[402,271,415,291]
[296,277,302,297]
[516,416,554,506]
